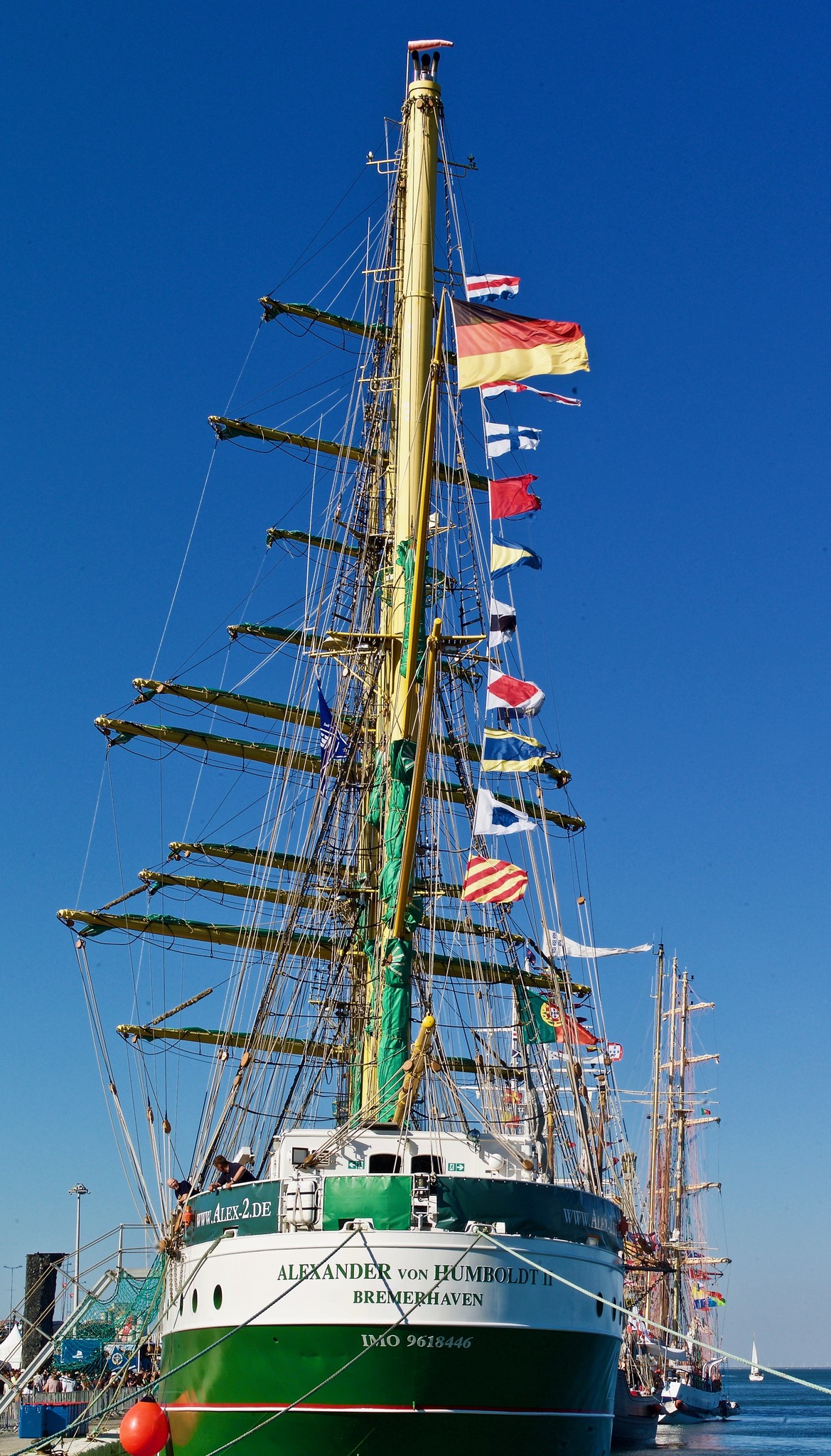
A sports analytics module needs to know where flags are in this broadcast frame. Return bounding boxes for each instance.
[473,787,538,836]
[486,668,545,719]
[449,271,590,406]
[480,726,547,773]
[489,474,542,521]
[460,853,529,904]
[501,929,727,1346]
[489,598,517,649]
[485,422,542,459]
[490,534,543,581]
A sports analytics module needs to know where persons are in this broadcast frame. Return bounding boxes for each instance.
[167,1177,198,1206]
[206,1155,257,1191]
[0,1366,160,1424]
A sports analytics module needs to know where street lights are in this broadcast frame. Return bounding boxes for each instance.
[2,1264,24,1337]
[68,1182,91,1338]
[59,1262,87,1330]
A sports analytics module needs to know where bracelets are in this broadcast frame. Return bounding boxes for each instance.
[232,1179,235,1183]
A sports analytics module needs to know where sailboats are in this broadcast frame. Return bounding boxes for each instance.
[56,28,673,1456]
[605,935,737,1427]
[748,1333,766,1381]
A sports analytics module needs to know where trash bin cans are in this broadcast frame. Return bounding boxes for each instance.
[18,1401,89,1438]
